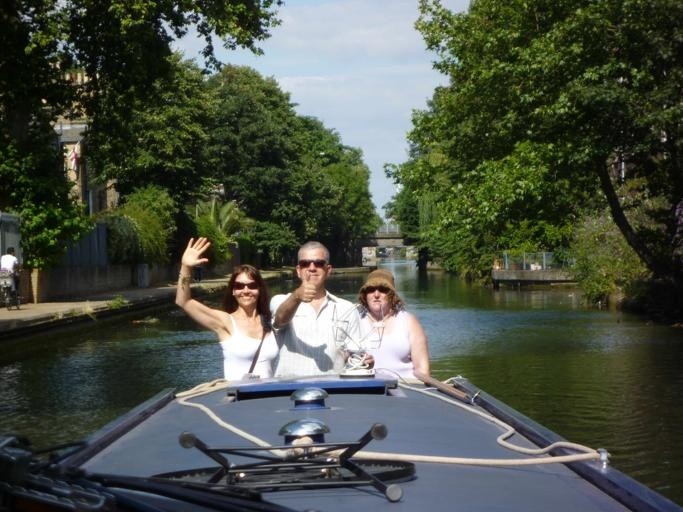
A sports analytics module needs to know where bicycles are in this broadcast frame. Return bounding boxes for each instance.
[2,270,24,310]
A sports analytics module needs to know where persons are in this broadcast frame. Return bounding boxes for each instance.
[520,258,531,270]
[353,268,431,384]
[269,241,376,376]
[174,235,283,382]
[0,246,24,300]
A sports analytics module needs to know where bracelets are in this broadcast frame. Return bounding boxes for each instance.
[177,271,194,287]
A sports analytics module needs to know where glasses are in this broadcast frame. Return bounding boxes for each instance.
[296,259,327,268]
[362,285,393,295]
[232,281,258,291]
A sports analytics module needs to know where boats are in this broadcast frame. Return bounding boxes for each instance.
[2,372,683,512]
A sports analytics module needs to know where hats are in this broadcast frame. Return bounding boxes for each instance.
[360,270,395,295]
